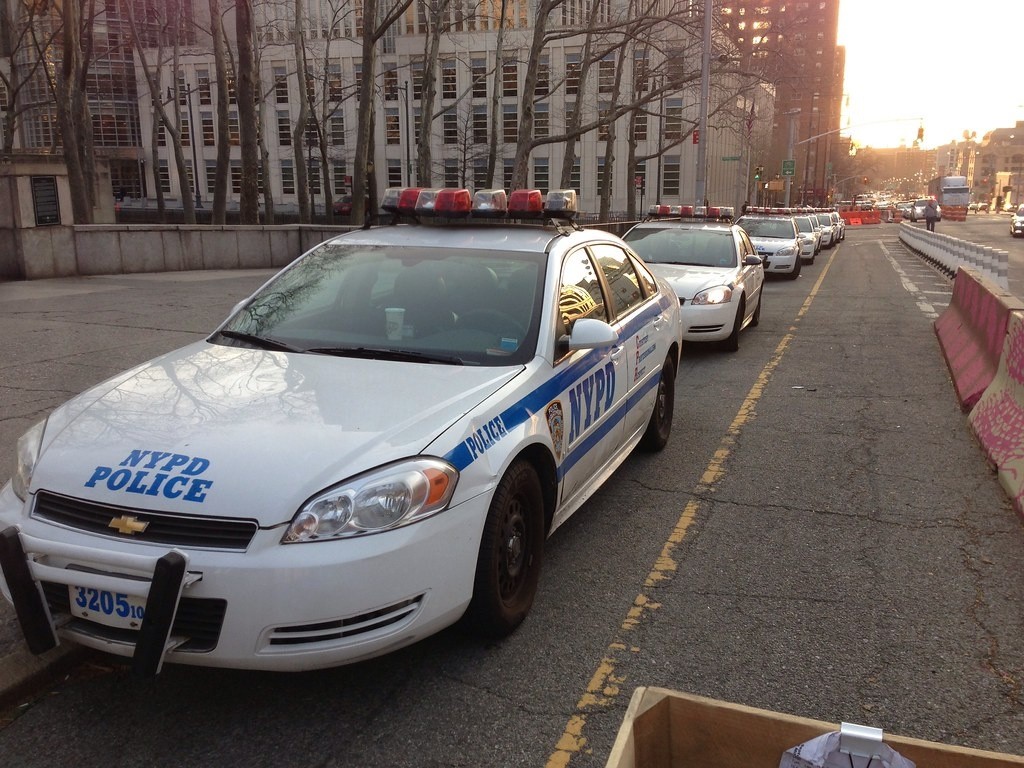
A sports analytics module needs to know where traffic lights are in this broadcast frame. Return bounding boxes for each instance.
[755,167,760,180]
[983,177,988,184]
[917,127,924,138]
[864,177,868,184]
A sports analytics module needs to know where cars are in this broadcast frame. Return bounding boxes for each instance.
[969,204,977,210]
[0,184,685,675]
[1010,204,1024,238]
[832,189,922,219]
[910,198,942,222]
[792,207,822,266]
[334,194,368,216]
[977,203,989,211]
[733,207,807,280]
[613,205,767,354]
[804,206,847,251]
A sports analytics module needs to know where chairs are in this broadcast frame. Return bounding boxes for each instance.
[374,271,456,339]
[445,265,501,315]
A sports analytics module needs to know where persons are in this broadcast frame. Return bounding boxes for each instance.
[704,196,709,206]
[741,201,748,213]
[925,200,936,232]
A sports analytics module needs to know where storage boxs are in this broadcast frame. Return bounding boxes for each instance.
[603,645,1024,768]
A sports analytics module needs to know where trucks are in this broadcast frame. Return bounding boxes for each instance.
[926,176,970,221]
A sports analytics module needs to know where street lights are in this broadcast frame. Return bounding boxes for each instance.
[165,83,204,208]
[650,74,661,206]
[388,80,411,188]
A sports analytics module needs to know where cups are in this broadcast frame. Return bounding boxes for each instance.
[385,307,405,340]
